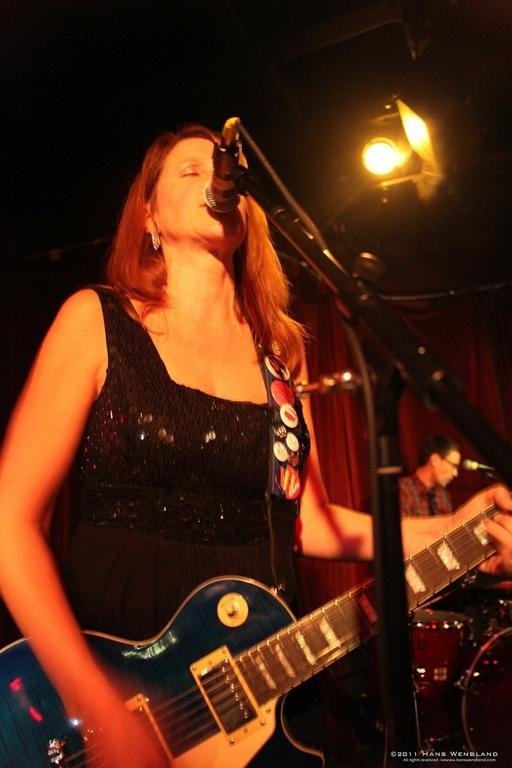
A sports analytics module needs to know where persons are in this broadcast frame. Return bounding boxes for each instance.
[1,122,512,767]
[395,434,464,519]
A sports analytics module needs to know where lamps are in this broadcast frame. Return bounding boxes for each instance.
[359,88,445,178]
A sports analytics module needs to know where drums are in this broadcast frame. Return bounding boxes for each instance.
[411,609,512,767]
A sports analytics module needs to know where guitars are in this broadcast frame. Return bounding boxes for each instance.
[2,503,500,766]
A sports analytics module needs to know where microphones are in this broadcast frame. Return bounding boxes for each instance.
[203,141,241,212]
[462,458,495,473]
